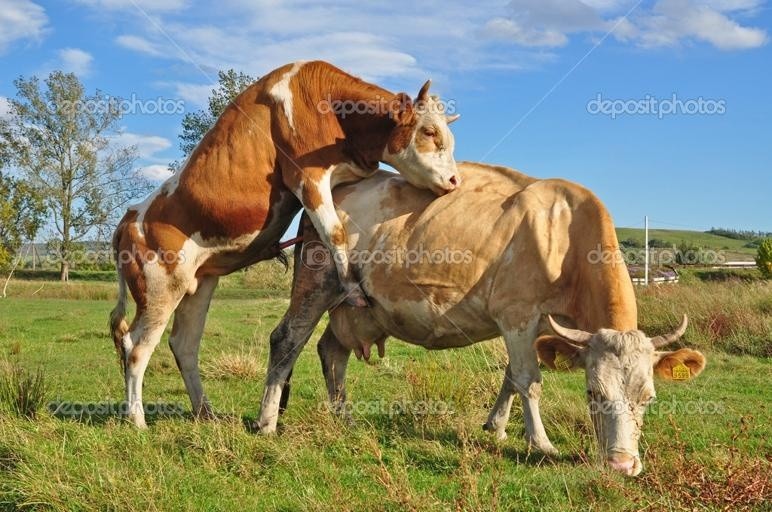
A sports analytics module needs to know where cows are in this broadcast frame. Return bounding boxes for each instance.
[253,160,707,477]
[106,59,461,429]
